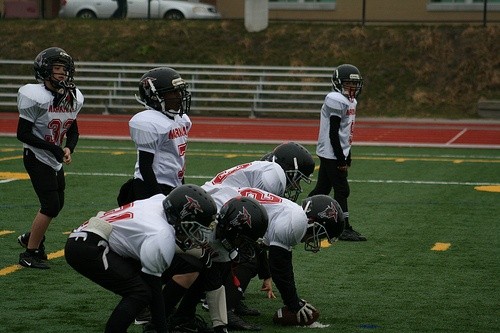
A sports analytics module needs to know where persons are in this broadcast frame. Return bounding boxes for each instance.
[65,142,345,333]
[17,49,84,269]
[309,65,366,240]
[116,68,192,207]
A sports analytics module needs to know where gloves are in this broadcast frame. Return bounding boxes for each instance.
[287,296,317,324]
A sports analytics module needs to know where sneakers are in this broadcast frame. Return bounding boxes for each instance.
[234,305,261,316]
[168,308,180,321]
[170,316,214,333]
[19,252,50,268]
[341,229,366,241]
[227,313,260,332]
[134,306,152,324]
[17,232,47,260]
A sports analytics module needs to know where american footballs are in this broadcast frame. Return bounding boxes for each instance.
[273,306,318,326]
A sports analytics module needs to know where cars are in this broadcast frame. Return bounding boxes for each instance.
[58,0,221,22]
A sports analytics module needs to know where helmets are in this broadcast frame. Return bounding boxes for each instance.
[273,142,315,182]
[332,63,362,93]
[162,184,217,229]
[220,196,268,247]
[33,47,76,82]
[302,195,345,240]
[139,67,186,109]
